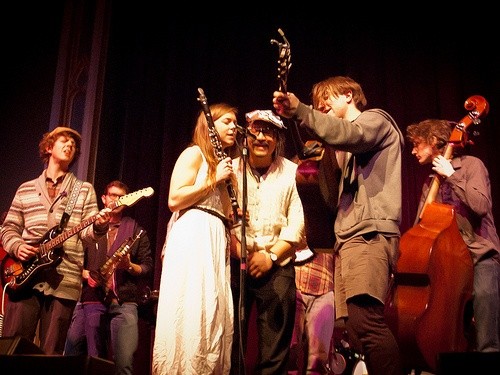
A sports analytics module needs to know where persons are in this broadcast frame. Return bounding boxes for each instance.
[65,74,500,375]
[0,127,111,355]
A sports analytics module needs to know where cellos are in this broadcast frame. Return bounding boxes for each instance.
[384,95,489,374]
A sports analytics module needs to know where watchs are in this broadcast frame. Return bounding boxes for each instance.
[266,250,278,261]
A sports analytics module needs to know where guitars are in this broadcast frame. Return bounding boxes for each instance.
[270,27,342,255]
[1,183,155,294]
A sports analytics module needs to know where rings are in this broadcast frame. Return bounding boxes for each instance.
[278,98,283,103]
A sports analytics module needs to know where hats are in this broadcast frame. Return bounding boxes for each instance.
[48,127,81,144]
[245,110,284,129]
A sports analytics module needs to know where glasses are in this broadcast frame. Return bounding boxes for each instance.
[247,126,275,136]
[106,193,124,200]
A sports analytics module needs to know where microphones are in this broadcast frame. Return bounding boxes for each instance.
[236,124,257,140]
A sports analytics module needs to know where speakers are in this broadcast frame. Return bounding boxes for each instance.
[0,335,117,375]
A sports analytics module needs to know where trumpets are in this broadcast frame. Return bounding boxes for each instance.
[89,229,144,290]
